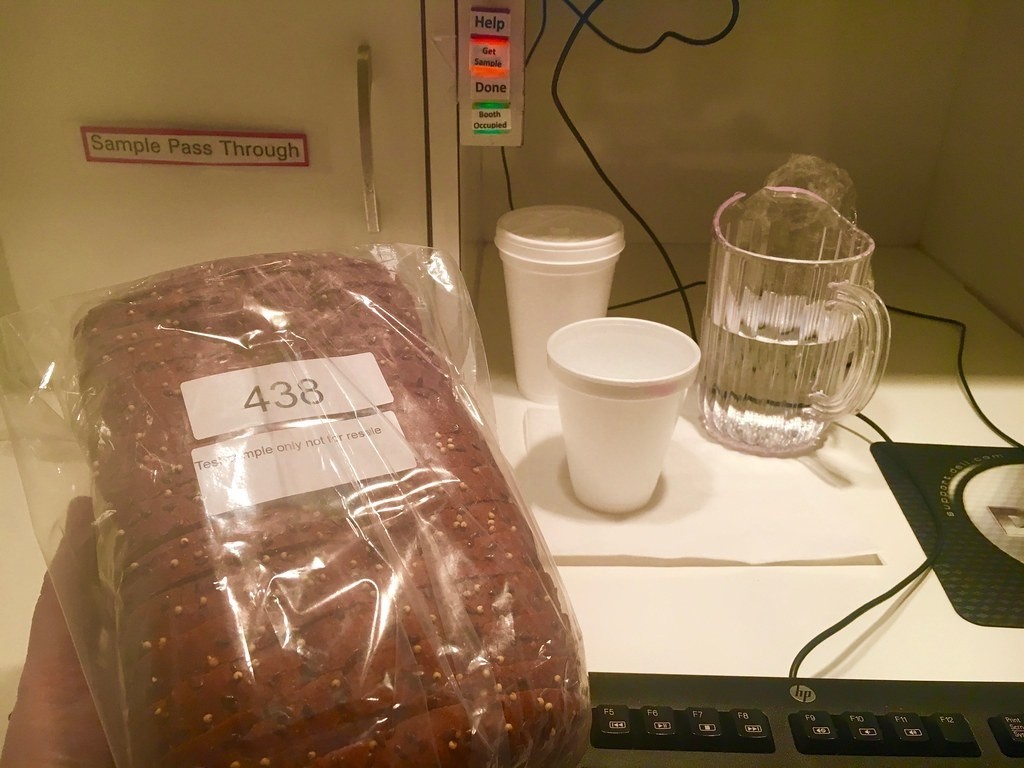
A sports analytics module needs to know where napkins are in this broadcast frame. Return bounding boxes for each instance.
[521,409,881,562]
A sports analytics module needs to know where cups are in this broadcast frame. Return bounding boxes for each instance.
[546,318,701,513]
[494,205,625,404]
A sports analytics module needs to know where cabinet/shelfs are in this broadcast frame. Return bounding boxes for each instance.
[0,0,482,386]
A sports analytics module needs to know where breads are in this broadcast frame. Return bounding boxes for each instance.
[73,253,593,768]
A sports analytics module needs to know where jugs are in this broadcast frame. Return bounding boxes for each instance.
[700,187,890,453]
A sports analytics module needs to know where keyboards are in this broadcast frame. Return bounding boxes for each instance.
[575,673,1024,768]
